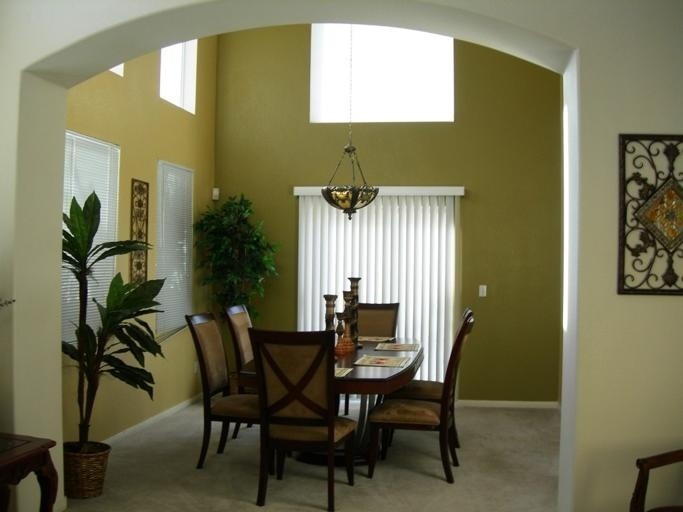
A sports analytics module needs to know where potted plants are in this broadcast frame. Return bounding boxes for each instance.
[61,188,166,498]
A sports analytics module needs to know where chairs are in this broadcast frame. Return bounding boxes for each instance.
[185,302,476,511]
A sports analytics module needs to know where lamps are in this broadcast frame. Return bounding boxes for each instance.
[322,21,379,221]
